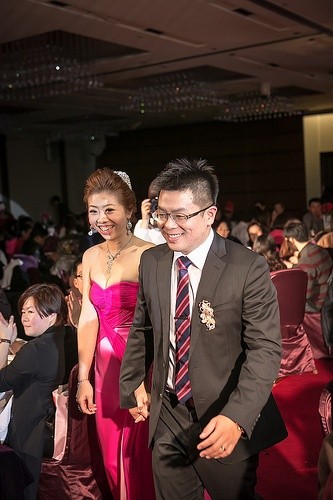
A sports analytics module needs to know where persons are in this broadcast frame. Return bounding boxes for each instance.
[119,158,289,500]
[0,187,143,357]
[0,284,78,500]
[211,191,333,317]
[76,167,211,500]
[134,174,167,245]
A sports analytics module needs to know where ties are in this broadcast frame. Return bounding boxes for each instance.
[173,256,194,405]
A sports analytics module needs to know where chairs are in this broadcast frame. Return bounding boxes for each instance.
[269,269,316,384]
[302,312,330,362]
[37,362,113,500]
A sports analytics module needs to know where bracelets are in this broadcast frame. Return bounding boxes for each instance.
[0,338,11,344]
[77,379,88,388]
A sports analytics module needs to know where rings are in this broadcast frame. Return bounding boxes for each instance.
[78,405,83,413]
[221,446,226,451]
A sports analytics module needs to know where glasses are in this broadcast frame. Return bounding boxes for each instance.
[76,274,83,280]
[156,203,213,224]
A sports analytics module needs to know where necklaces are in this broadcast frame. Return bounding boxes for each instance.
[104,234,134,288]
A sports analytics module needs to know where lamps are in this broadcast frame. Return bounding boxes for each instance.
[214,89,302,122]
[0,31,103,100]
[119,71,229,114]
